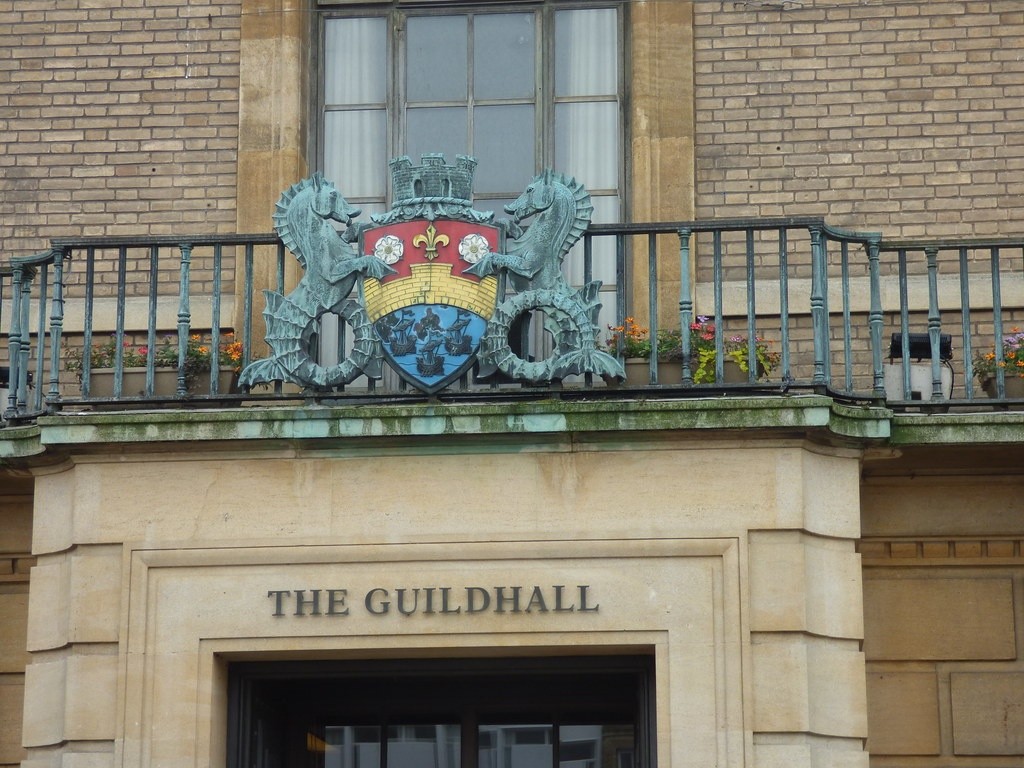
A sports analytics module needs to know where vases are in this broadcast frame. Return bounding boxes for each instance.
[76,364,244,408]
[978,371,1024,411]
[606,356,765,387]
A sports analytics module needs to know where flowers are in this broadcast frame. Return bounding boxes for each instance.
[63,328,247,368]
[972,326,1024,385]
[606,315,781,380]
